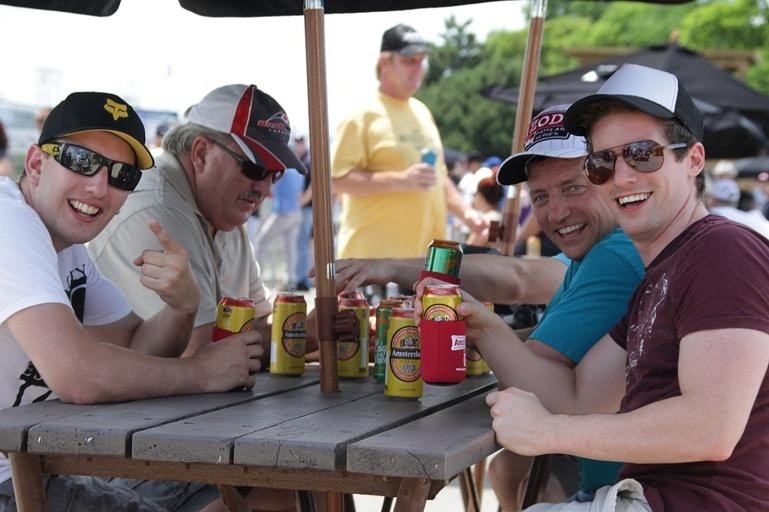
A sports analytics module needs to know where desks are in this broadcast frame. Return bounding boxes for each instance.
[0,363,542,511]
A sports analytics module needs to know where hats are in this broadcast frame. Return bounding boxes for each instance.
[188,83,308,175]
[497,102,587,186]
[39,91,154,170]
[563,63,703,138]
[381,26,435,57]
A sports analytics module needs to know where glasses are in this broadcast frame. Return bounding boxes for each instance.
[41,142,142,191]
[583,140,688,184]
[205,136,284,184]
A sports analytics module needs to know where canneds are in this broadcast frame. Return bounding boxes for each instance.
[423,238,463,273]
[269,289,306,378]
[422,284,463,321]
[466,300,495,376]
[337,291,367,299]
[384,307,424,398]
[213,297,255,342]
[374,301,409,382]
[338,298,369,377]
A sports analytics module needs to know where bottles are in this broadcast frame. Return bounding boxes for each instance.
[511,236,548,330]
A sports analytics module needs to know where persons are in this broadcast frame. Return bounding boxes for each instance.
[1,80,358,512]
[331,25,487,260]
[305,58,768,510]
